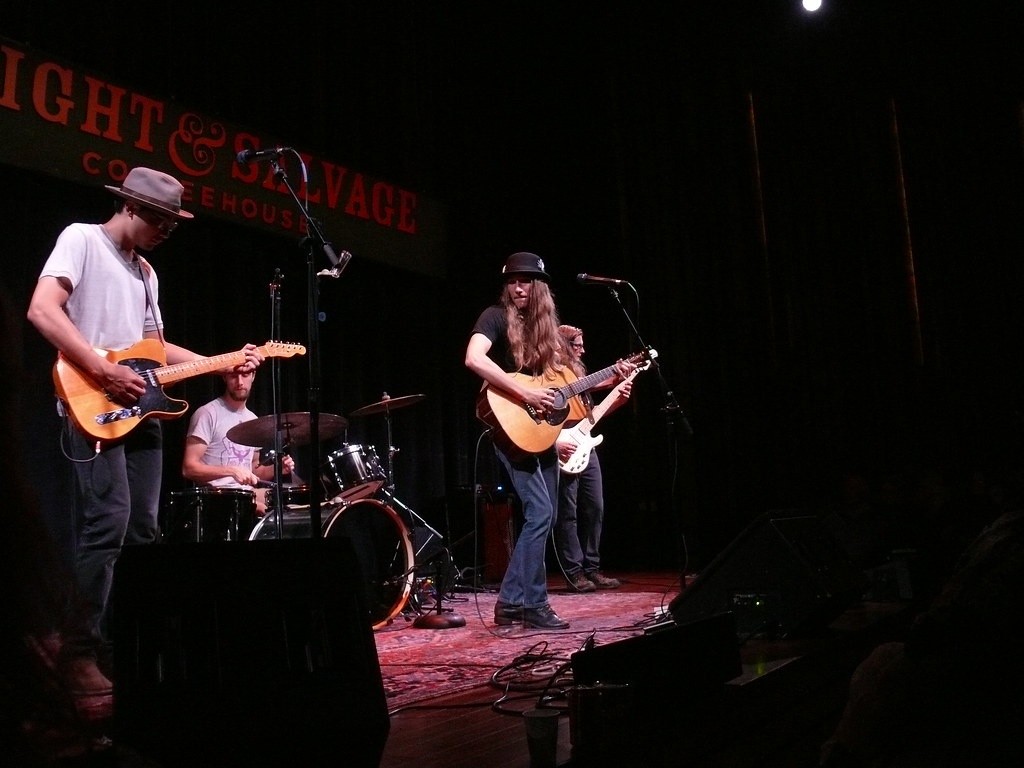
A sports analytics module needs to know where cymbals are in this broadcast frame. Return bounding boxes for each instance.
[226,411,351,449]
[351,392,427,417]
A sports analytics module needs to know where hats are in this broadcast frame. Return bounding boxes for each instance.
[104,167,195,218]
[500,252,550,280]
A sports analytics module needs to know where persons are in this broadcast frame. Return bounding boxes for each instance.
[181,346,295,516]
[27,167,265,697]
[545,325,633,593]
[465,253,637,630]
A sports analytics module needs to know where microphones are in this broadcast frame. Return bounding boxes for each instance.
[238,147,291,164]
[576,274,629,287]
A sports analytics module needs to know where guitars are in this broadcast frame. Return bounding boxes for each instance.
[473,348,659,465]
[52,338,308,448]
[555,359,652,476]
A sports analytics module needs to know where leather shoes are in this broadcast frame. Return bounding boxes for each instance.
[493,599,526,625]
[520,606,570,630]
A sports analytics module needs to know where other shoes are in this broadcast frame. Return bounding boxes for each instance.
[25,626,63,673]
[58,656,115,695]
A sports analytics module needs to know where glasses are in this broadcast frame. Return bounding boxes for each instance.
[570,341,585,350]
[133,207,177,232]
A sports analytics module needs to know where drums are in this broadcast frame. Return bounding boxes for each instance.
[317,442,388,503]
[165,486,257,548]
[248,498,417,634]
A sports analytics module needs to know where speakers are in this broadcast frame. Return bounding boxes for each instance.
[111,533,391,768]
[411,527,442,577]
[480,495,527,584]
[668,509,860,642]
[570,610,740,726]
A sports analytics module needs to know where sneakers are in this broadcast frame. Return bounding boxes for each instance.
[566,570,598,591]
[586,570,619,589]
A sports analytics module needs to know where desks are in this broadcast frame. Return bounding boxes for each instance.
[112,536,391,768]
